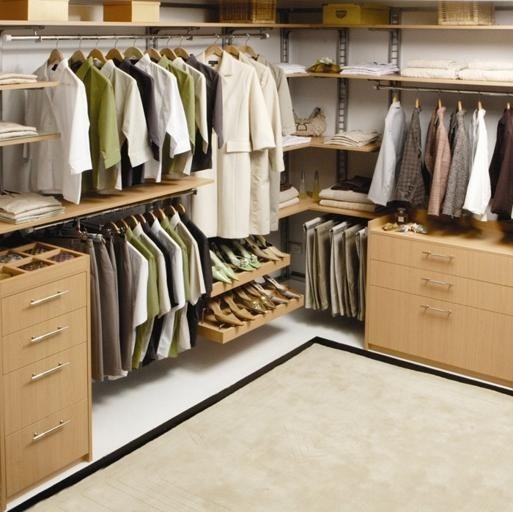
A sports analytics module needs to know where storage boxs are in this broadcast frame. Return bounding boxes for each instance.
[102,1,161,23]
[321,1,390,25]
[0,1,69,22]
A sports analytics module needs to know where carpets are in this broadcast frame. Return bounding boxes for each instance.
[9,343,512,512]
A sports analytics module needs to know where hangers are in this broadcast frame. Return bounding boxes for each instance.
[30,194,186,243]
[393,85,511,114]
[46,30,260,66]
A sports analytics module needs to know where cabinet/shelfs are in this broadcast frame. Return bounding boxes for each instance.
[1,241,92,478]
[364,207,513,389]
[1,0,512,236]
[198,246,304,345]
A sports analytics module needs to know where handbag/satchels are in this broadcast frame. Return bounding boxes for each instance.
[291,108,326,137]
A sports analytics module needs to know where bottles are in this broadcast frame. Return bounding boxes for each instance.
[299,171,307,200]
[395,208,408,224]
[312,170,321,202]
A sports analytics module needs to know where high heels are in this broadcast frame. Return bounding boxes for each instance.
[233,286,266,314]
[208,249,239,281]
[249,280,288,304]
[244,237,281,261]
[206,297,244,326]
[243,283,276,310]
[210,265,232,284]
[219,244,256,271]
[253,234,289,258]
[231,240,262,268]
[262,274,301,298]
[221,293,255,320]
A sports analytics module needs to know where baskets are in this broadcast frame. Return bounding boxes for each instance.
[437,1,495,26]
[218,1,277,24]
